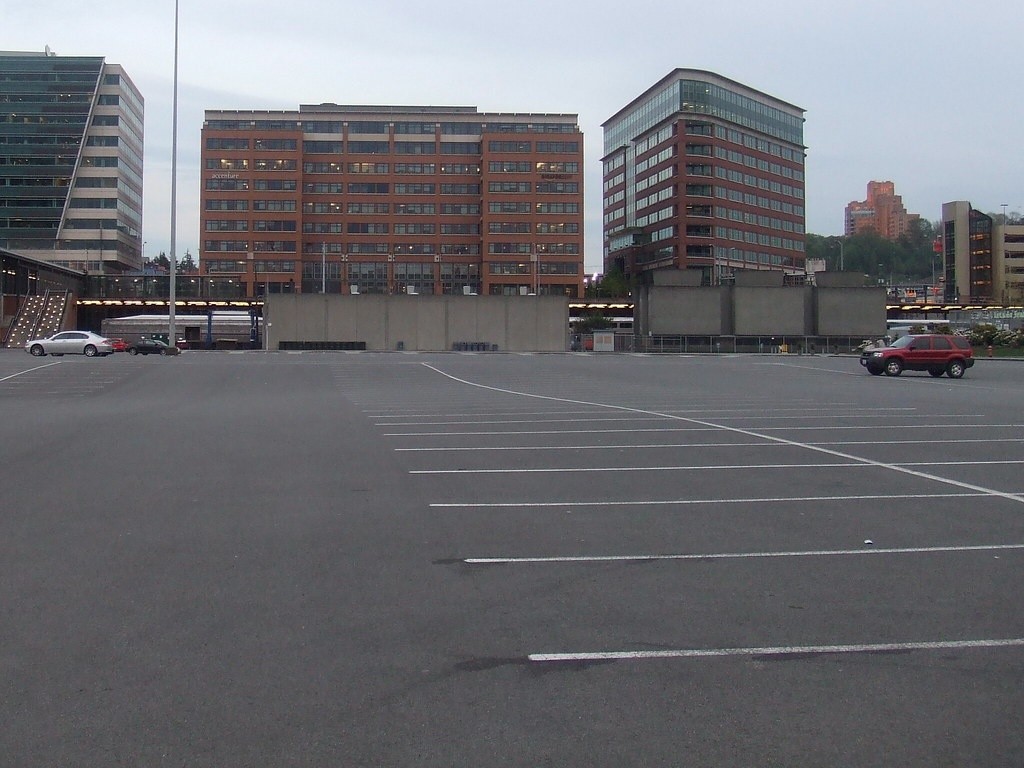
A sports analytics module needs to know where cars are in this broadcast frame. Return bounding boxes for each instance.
[108,338,129,353]
[125,337,182,356]
[175,339,192,350]
[23,330,114,357]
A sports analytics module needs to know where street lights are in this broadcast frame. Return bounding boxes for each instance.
[1000,204,1009,225]
[142,241,146,291]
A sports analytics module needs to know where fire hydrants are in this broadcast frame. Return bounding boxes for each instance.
[987,345,993,357]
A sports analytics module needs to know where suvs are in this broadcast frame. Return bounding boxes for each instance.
[859,334,976,378]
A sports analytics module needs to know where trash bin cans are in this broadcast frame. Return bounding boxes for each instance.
[492,343,498,351]
[471,341,478,351]
[396,341,404,351]
[484,342,490,351]
[453,341,461,351]
[460,341,467,351]
[478,341,484,351]
[466,342,472,351]
